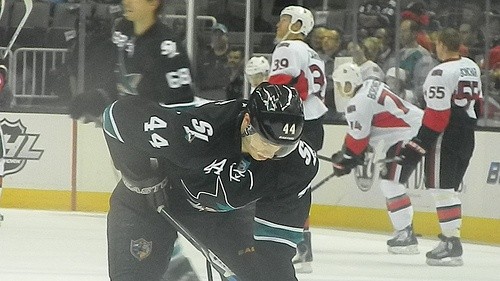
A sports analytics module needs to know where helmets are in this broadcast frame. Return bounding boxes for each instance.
[243,56,270,76]
[246,82,305,146]
[331,63,363,95]
[280,5,314,36]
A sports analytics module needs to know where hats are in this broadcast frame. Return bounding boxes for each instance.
[212,23,227,33]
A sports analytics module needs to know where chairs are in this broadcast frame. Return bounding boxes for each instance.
[0,0,180,48]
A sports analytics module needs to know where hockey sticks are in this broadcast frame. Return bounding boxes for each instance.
[317,155,334,163]
[310,174,336,192]
[377,155,405,164]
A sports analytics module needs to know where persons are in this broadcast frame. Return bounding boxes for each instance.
[0,58,13,221]
[103,81,319,281]
[331,61,426,246]
[67,0,200,281]
[196,0,500,121]
[265,6,327,263]
[396,29,484,260]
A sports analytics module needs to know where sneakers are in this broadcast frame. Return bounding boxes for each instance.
[424,233,463,266]
[386,226,420,254]
[291,231,313,273]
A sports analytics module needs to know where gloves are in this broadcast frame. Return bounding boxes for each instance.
[397,136,430,165]
[331,150,354,176]
[129,186,165,214]
[68,89,109,125]
